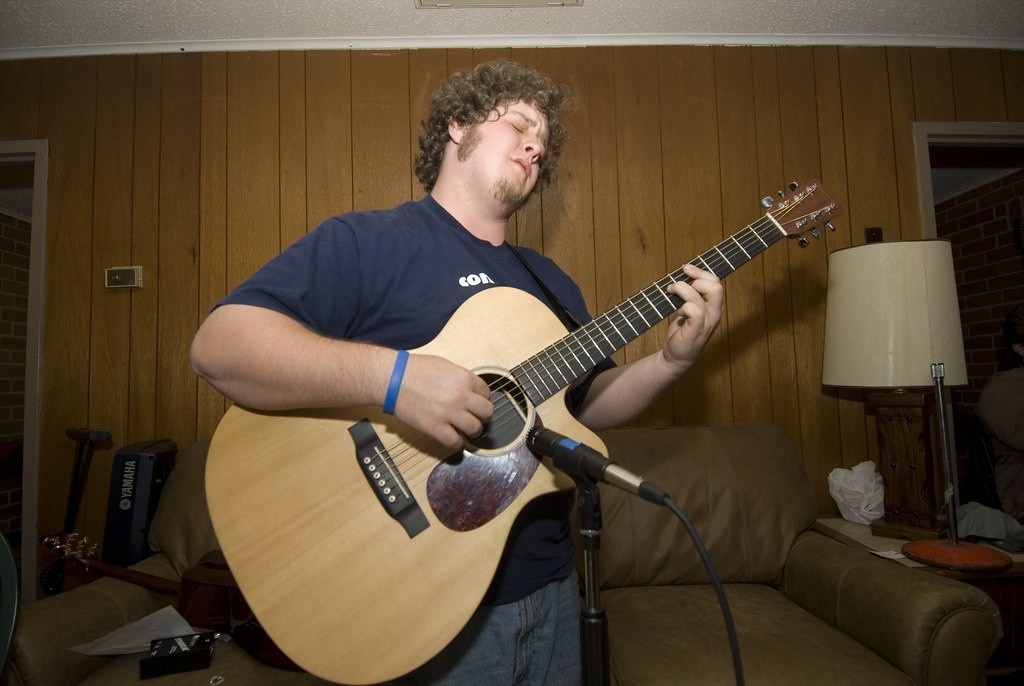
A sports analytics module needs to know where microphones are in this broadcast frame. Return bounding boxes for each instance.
[525,425,670,506]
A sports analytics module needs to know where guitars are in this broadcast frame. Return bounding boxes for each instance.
[203,175,846,686]
[40,527,303,672]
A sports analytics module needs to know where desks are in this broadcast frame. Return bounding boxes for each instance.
[815,516,1024,677]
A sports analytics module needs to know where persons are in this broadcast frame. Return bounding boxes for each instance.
[977,303,1024,526]
[190,56,724,686]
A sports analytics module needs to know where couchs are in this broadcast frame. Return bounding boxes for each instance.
[0,417,1009,686]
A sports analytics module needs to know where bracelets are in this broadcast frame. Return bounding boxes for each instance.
[383,350,409,415]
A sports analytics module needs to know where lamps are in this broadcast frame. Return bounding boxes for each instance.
[818,238,970,539]
